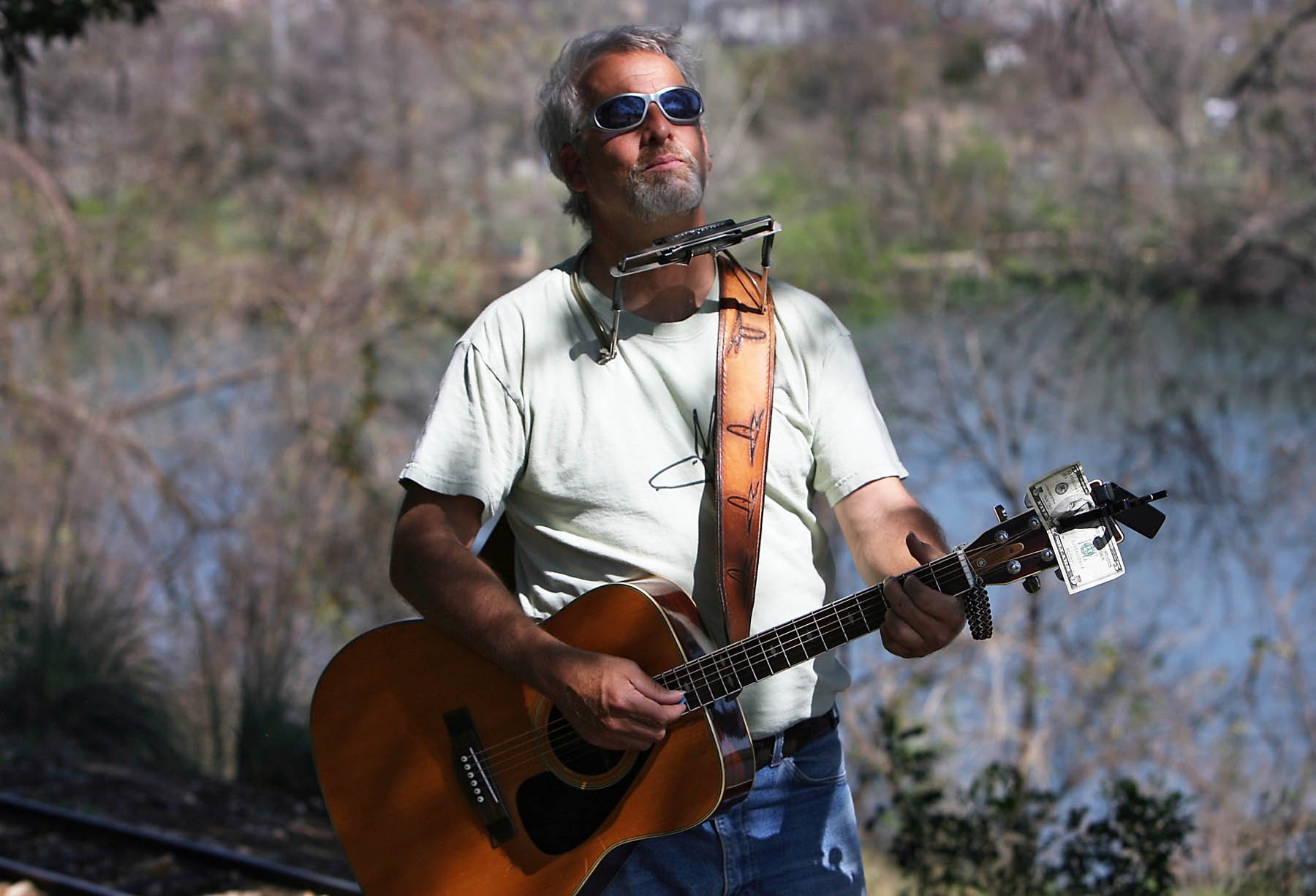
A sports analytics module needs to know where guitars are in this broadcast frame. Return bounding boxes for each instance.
[308,459,1173,896]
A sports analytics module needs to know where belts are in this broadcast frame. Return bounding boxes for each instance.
[752,704,840,772]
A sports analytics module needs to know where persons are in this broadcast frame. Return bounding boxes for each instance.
[390,22,965,896]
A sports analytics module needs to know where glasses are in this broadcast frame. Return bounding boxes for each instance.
[577,85,705,133]
